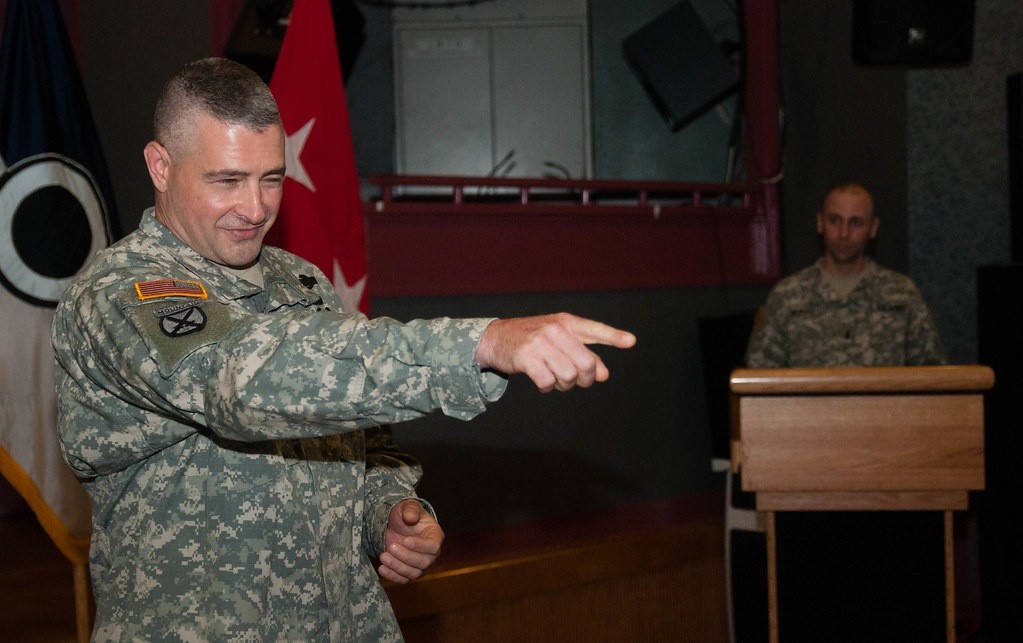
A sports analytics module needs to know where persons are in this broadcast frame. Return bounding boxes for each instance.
[729,181,953,643]
[48,56,637,643]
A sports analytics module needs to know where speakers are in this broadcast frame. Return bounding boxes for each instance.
[621,0,738,136]
[850,0,977,70]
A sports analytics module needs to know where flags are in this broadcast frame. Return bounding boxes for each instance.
[0,0,125,643]
[260,0,371,320]
